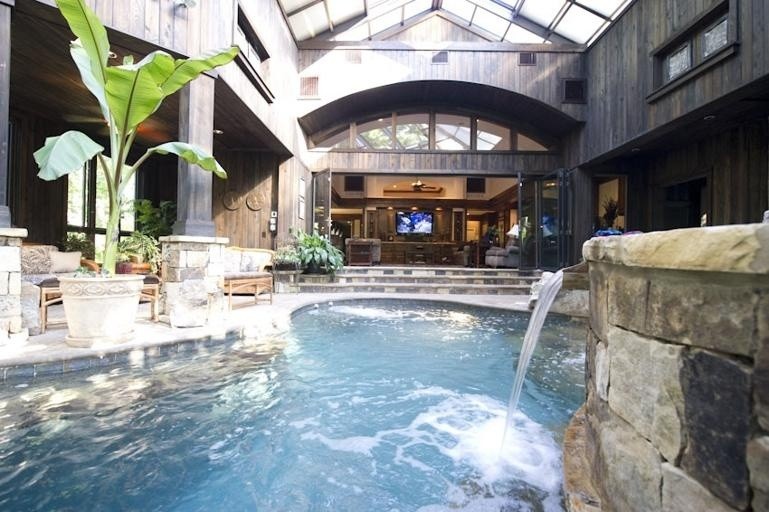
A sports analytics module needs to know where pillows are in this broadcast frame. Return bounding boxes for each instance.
[48,250,83,275]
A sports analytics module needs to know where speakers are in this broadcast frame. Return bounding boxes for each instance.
[344,176,364,192]
[467,177,485,193]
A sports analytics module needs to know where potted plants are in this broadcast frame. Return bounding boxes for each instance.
[117,229,164,274]
[30,0,242,344]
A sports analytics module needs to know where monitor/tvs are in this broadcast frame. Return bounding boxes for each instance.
[395,211,434,237]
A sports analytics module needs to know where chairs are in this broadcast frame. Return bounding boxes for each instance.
[485,237,528,268]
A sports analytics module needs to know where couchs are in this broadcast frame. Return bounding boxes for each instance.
[20,242,101,302]
[224,246,276,295]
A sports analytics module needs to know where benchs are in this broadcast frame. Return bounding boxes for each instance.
[221,271,273,313]
[36,276,160,335]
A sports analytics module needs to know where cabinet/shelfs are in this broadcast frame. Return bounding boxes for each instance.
[348,241,373,267]
